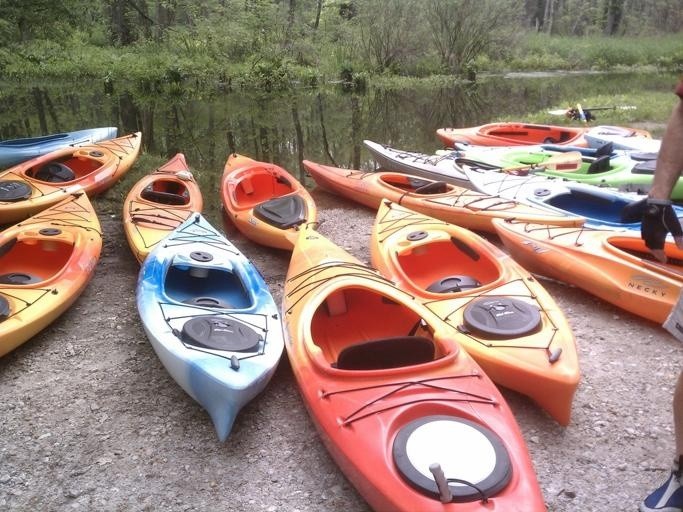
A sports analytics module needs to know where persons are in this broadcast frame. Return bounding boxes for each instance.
[643,82,683,511]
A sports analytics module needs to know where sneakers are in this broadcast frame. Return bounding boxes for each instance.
[640,463,683,512]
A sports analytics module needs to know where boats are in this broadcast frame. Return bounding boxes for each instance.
[220,153,317,252]
[0,125,142,223]
[136,211,284,442]
[0,190,102,355]
[123,151,203,265]
